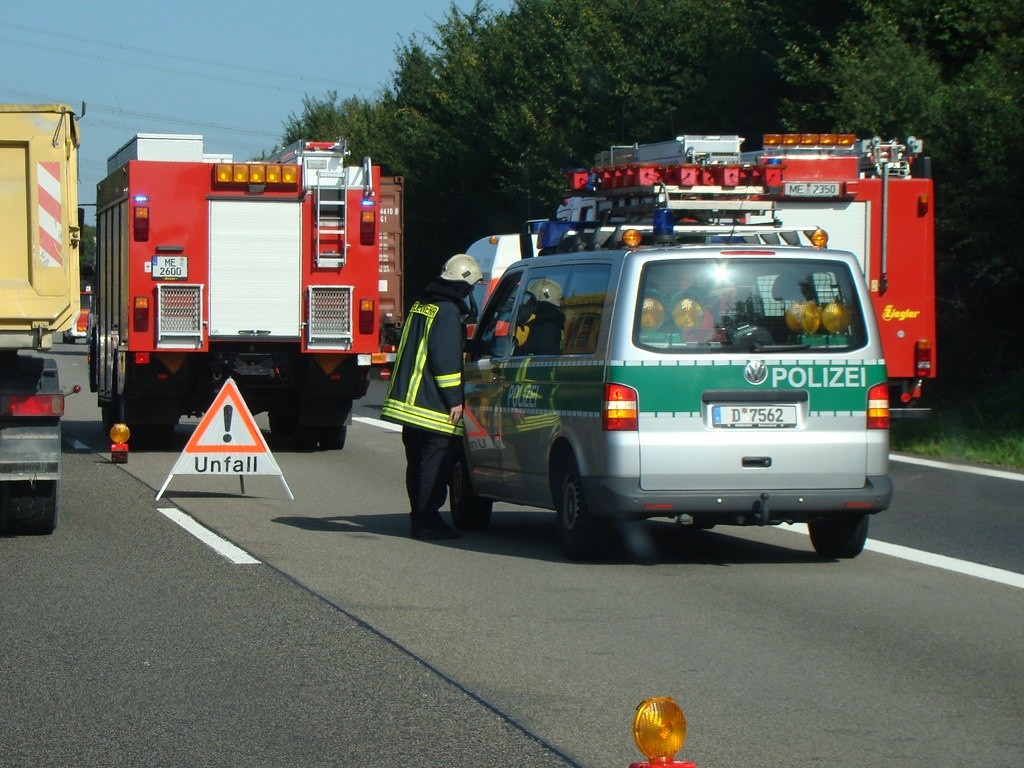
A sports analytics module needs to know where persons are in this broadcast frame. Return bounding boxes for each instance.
[382,254,482,537]
[502,278,566,489]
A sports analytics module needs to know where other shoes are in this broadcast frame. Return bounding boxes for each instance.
[410,518,463,539]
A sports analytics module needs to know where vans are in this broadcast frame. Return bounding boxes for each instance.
[466,162,894,564]
[63,291,94,343]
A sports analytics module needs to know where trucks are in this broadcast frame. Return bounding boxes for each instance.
[379,175,407,334]
[1,104,82,538]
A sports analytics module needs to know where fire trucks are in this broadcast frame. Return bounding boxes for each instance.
[79,134,389,450]
[460,132,941,422]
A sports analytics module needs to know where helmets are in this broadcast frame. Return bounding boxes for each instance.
[440,254,484,286]
[526,279,563,307]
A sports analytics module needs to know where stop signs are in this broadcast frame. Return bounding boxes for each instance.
[381,368,392,380]
